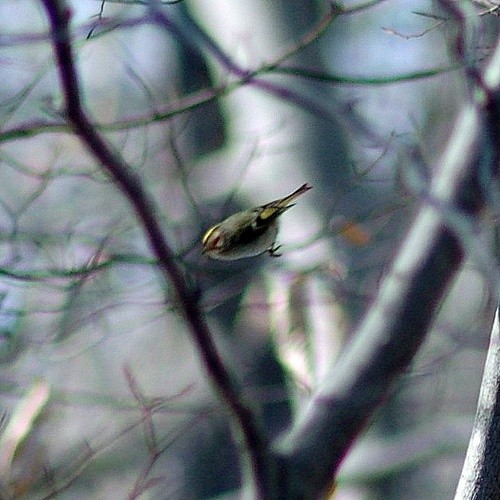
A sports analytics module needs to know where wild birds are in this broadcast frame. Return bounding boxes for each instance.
[200,181,314,259]
